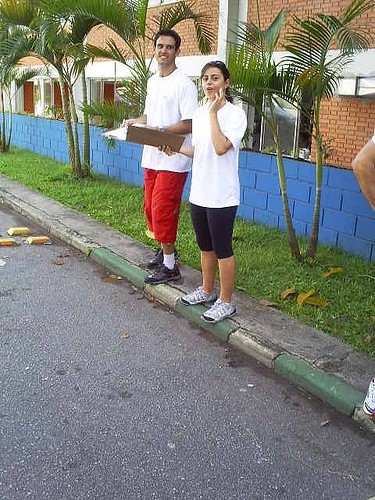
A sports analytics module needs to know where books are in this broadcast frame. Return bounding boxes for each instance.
[100,122,186,154]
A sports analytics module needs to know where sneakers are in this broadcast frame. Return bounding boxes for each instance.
[362,374,375,416]
[144,247,181,285]
[179,286,236,324]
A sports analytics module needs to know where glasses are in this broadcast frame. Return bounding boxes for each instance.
[215,60,227,69]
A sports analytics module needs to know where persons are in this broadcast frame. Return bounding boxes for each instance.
[123,29,198,285]
[158,60,246,324]
[350,129,375,415]
